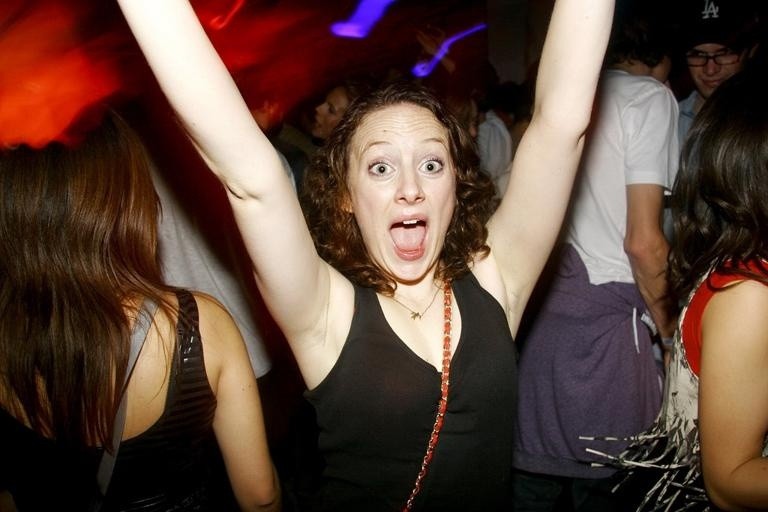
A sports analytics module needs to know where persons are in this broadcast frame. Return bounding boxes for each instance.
[246,68,358,184]
[117,1,614,512]
[1,115,280,511]
[415,1,768,512]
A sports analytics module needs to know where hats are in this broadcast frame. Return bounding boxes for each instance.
[682,1,759,48]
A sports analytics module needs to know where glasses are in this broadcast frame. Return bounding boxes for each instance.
[681,45,746,68]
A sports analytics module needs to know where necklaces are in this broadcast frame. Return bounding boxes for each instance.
[393,283,442,321]
